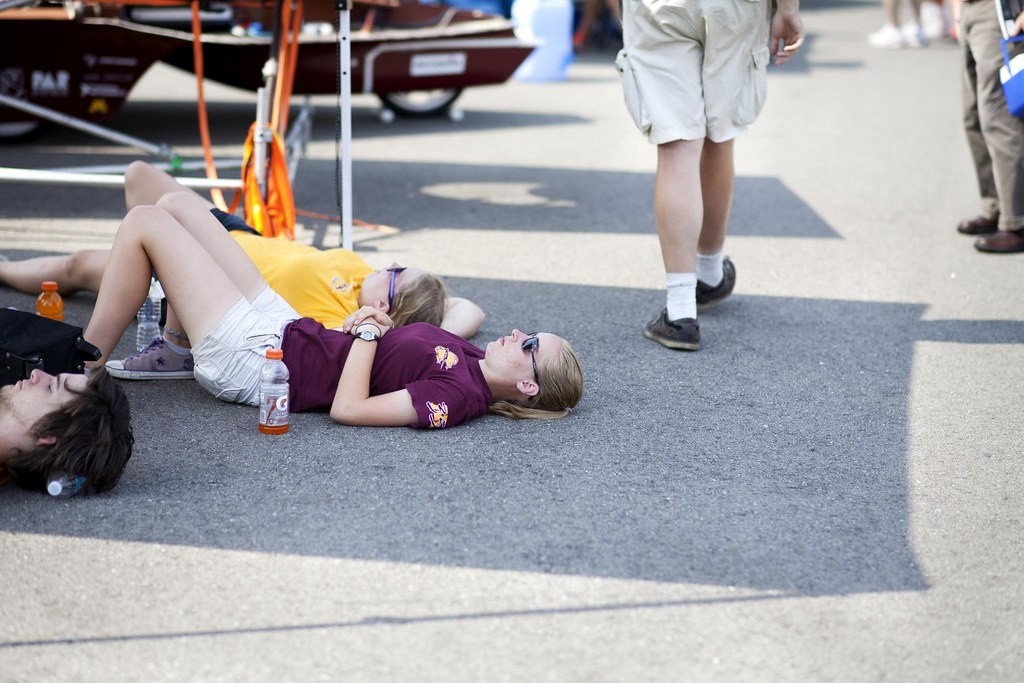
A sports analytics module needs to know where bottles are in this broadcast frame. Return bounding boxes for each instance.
[36,280,64,321]
[259,348,290,434]
[136,272,161,353]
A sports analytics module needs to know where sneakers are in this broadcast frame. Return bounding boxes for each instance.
[104,338,195,380]
[643,306,701,350]
[696,258,736,309]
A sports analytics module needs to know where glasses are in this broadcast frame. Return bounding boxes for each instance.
[387,268,407,307]
[521,332,540,390]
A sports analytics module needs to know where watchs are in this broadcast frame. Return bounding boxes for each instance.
[352,330,379,342]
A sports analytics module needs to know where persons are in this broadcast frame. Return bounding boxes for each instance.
[867,0,959,49]
[574,0,623,53]
[79,190,584,431]
[956,0,1024,254]
[0,160,486,340]
[0,367,136,495]
[616,0,806,350]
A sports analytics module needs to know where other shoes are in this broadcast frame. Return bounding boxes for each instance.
[919,2,958,41]
[868,21,928,49]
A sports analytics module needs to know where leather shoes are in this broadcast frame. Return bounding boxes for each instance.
[958,210,1000,235]
[974,228,1024,253]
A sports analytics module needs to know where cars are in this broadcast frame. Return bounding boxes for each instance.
[0,1,540,127]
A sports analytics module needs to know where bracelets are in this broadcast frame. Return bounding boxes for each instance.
[355,323,382,336]
